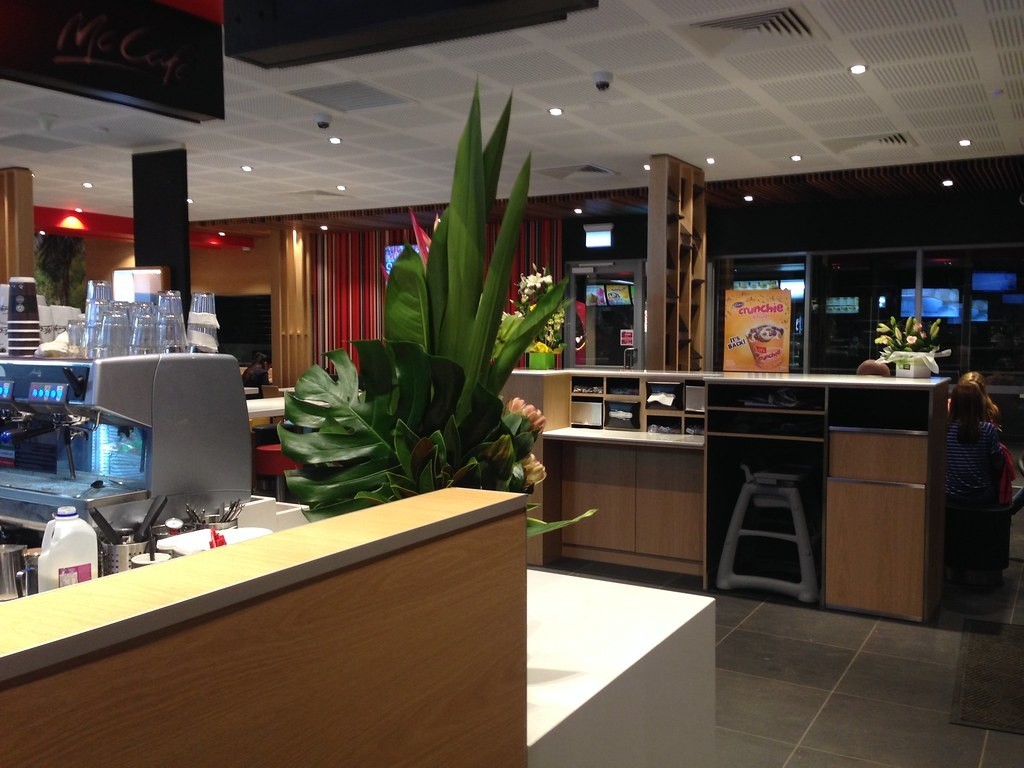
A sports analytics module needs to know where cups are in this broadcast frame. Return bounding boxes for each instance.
[67,279,189,360]
[0,277,41,357]
[745,322,785,370]
[36,305,54,344]
[131,553,172,577]
[186,322,219,353]
[188,290,220,328]
[51,304,73,341]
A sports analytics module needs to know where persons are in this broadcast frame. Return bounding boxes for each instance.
[945,372,1005,505]
[241,353,273,399]
[956,345,1018,385]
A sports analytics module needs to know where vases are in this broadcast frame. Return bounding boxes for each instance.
[529,353,555,370]
[895,359,935,378]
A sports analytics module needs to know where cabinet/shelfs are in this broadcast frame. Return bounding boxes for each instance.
[507,368,951,624]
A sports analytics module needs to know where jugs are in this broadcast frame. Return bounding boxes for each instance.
[0,544,28,601]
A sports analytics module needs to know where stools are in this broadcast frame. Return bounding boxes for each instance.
[715,451,820,605]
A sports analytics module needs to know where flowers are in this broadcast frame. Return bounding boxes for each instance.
[875,316,938,352]
[513,258,574,354]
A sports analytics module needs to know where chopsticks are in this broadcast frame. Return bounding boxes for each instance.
[185,501,198,523]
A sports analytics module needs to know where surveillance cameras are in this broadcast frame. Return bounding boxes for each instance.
[315,113,333,128]
[592,71,613,90]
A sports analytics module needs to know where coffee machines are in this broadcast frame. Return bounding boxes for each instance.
[0,353,252,543]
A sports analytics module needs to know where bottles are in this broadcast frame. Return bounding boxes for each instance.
[38,506,98,595]
[597,288,605,305]
